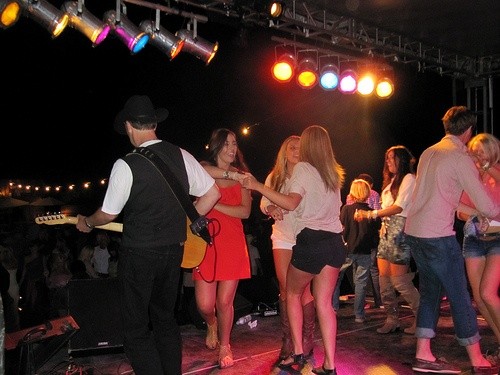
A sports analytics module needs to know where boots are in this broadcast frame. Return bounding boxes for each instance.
[301,297,316,358]
[390,273,424,335]
[276,296,297,359]
[376,272,401,335]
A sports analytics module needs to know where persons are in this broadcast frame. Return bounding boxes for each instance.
[182,233,280,324]
[403,105,500,375]
[75,95,222,375]
[241,124,348,375]
[260,135,316,365]
[0,231,121,375]
[456,133,500,360]
[333,173,383,323]
[192,128,253,370]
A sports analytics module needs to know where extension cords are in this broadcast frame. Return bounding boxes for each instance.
[254,310,277,316]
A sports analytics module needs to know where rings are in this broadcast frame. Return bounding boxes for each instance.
[272,214,280,217]
[355,208,359,217]
[354,145,420,335]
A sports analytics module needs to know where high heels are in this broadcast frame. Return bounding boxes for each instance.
[278,350,308,372]
[313,362,337,375]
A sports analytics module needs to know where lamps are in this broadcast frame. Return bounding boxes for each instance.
[237,0,286,19]
[0,1,220,65]
[270,40,396,100]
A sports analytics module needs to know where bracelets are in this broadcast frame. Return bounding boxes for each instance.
[372,210,378,221]
[232,170,237,181]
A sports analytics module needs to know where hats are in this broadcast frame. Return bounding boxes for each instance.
[111,94,169,123]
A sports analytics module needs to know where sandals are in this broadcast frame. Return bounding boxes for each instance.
[217,346,234,370]
[205,318,218,348]
[487,346,500,368]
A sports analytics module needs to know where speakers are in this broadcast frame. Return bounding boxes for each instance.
[186,291,252,330]
[66,278,124,353]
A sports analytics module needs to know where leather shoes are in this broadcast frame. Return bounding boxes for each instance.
[413,354,463,374]
[470,359,500,375]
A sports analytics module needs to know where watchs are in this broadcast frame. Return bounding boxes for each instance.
[223,170,231,180]
[367,211,371,221]
[482,160,491,170]
[84,216,95,230]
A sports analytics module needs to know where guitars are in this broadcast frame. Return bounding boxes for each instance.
[35,210,207,269]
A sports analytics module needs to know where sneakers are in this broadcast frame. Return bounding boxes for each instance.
[354,314,371,325]
